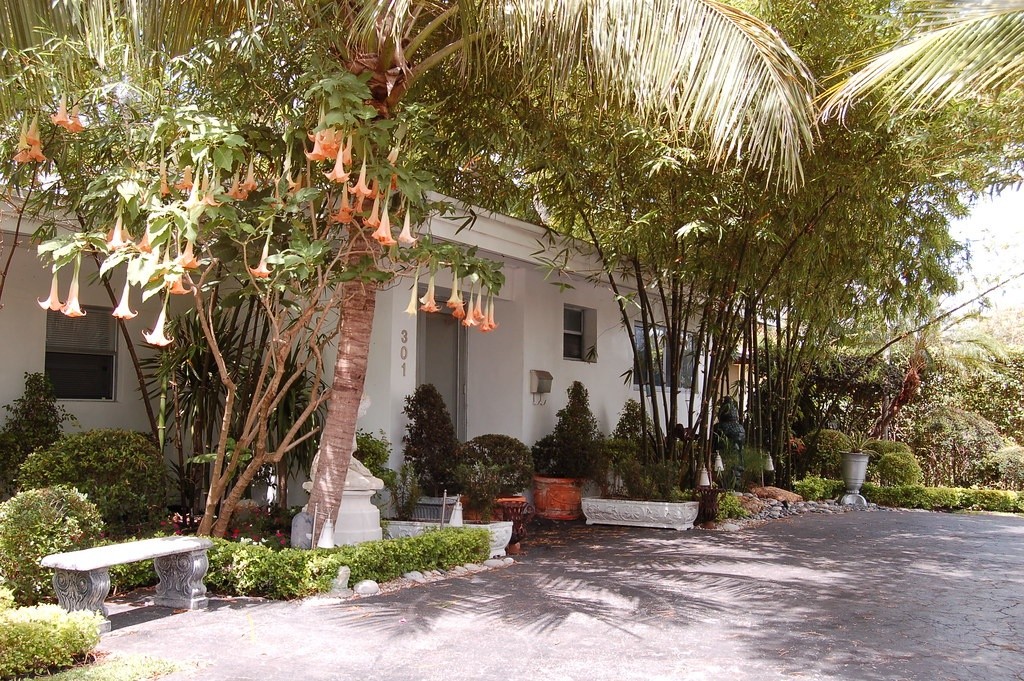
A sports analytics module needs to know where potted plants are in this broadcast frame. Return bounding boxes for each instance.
[534,436,595,520]
[401,383,469,522]
[460,433,534,546]
[581,398,702,526]
[835,430,881,506]
[552,380,604,501]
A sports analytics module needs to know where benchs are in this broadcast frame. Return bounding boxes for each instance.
[41,534,212,636]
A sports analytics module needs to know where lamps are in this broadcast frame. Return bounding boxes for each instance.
[700,464,710,486]
[714,450,725,479]
[764,452,775,471]
